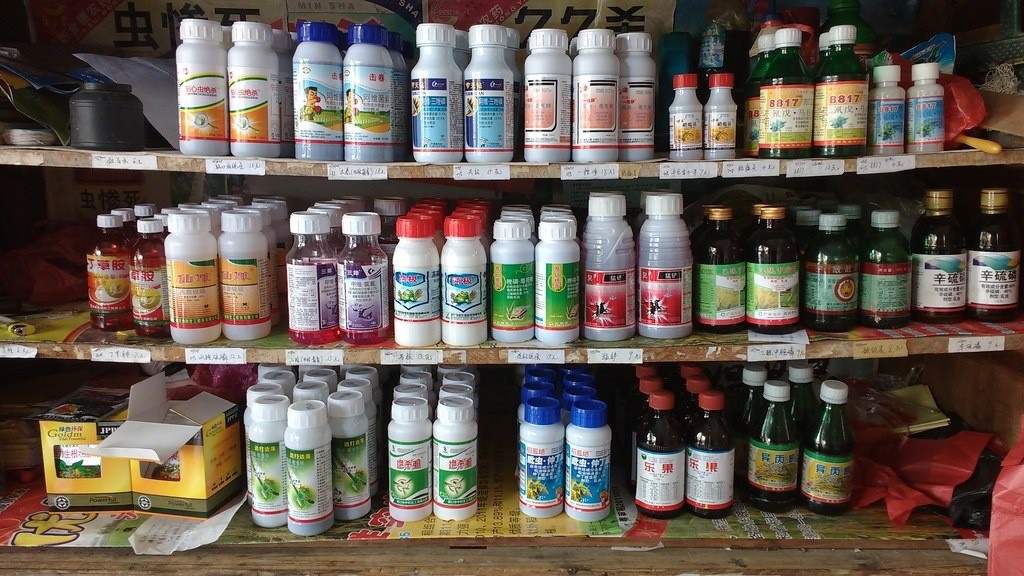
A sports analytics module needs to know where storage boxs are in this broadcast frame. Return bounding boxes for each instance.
[80,371,243,520]
[40,387,132,514]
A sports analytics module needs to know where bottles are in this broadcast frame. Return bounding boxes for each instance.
[740,364,855,516]
[517,364,612,522]
[704,73,737,160]
[177,18,657,165]
[243,363,477,536]
[631,364,735,520]
[669,74,702,161]
[744,25,946,158]
[87,188,1021,347]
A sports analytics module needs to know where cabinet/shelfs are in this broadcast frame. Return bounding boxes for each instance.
[0,146,1024,548]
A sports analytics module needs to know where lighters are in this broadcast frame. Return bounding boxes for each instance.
[0,314,36,338]
